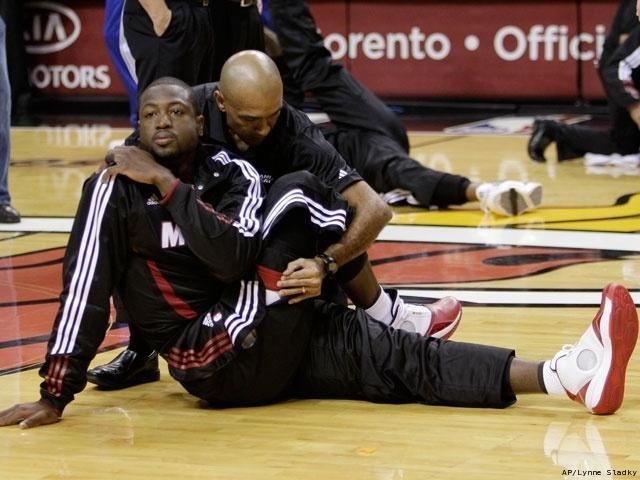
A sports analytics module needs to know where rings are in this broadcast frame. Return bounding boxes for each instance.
[302,286,306,294]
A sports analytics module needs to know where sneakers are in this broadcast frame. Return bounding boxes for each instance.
[383,287,463,340]
[478,181,544,217]
[548,282,639,416]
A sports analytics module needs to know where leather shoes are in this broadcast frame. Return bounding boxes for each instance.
[0,205,23,224]
[85,346,161,392]
[527,117,552,164]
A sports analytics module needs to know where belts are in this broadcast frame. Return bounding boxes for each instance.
[188,0,257,7]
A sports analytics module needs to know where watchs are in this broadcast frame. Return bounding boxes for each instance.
[316,252,338,277]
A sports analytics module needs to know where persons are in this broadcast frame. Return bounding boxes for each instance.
[0,76,639,435]
[0,0,639,222]
[88,46,392,391]
